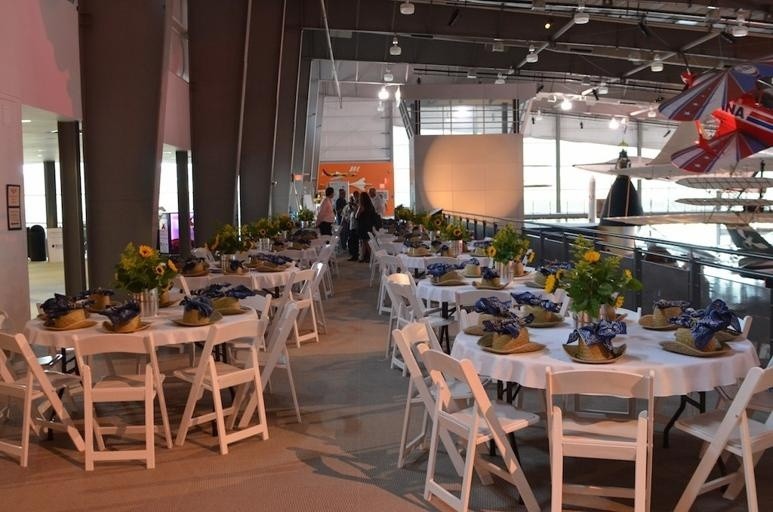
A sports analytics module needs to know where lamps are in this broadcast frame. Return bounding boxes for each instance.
[377,0,749,128]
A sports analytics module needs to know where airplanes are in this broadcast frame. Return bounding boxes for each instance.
[567,60,772,289]
[347,177,374,192]
[319,166,358,180]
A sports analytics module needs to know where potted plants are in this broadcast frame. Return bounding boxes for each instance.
[113,241,178,293]
[547,234,644,315]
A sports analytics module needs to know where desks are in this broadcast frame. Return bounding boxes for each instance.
[25,298,260,395]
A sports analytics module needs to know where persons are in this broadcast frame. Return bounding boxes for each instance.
[315,186,387,263]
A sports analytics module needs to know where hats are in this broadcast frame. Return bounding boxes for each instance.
[38,285,255,334]
[403,240,487,257]
[638,299,742,357]
[182,252,293,277]
[426,258,626,364]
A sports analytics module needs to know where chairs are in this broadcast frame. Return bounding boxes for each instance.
[672,353,773,511]
[417,343,542,512]
[224,297,303,428]
[391,318,490,477]
[664,309,753,434]
[174,315,272,454]
[173,218,561,378]
[543,366,654,511]
[72,329,173,470]
[1,311,86,468]
